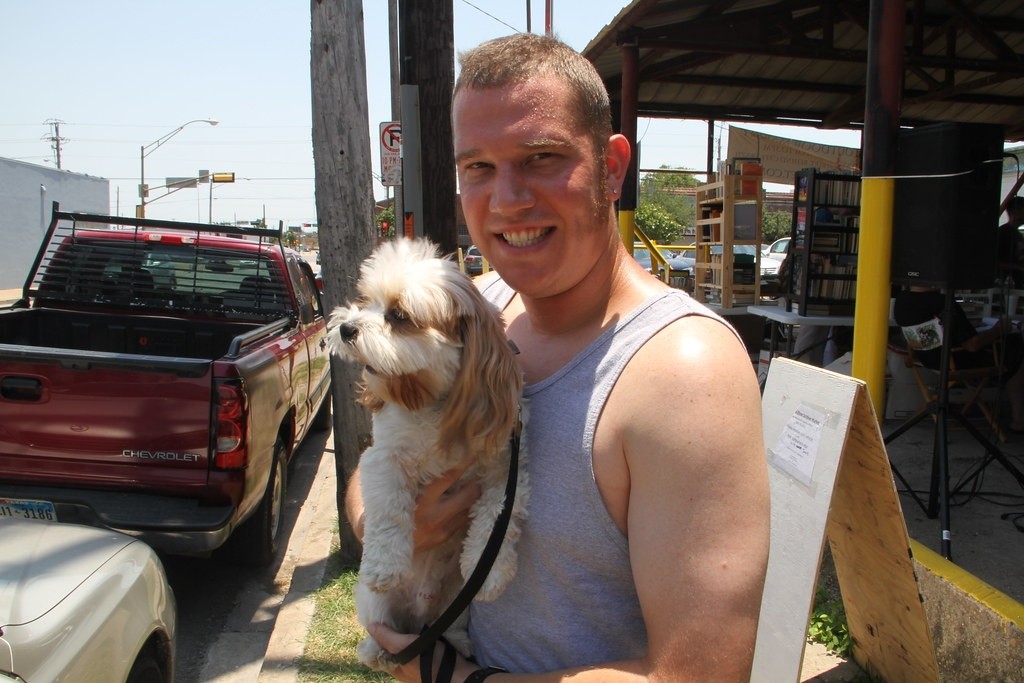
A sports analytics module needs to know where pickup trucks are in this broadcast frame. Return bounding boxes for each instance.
[0,201,332,574]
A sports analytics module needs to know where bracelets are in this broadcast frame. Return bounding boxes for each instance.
[464,668,509,683]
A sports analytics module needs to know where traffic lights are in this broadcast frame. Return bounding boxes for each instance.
[381,220,389,237]
[304,224,311,227]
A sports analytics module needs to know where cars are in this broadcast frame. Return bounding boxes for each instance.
[464,245,493,276]
[675,241,781,277]
[103,251,177,292]
[0,516,180,683]
[316,251,321,265]
[760,237,792,261]
[239,260,257,269]
[303,245,311,252]
[633,240,695,281]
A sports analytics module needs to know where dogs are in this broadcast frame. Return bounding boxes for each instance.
[325,234,532,671]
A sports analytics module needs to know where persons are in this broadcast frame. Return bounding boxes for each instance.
[894,284,1024,431]
[151,253,177,289]
[346,34,771,683]
[999,195,1024,279]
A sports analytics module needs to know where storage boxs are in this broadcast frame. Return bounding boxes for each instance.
[886,342,1000,424]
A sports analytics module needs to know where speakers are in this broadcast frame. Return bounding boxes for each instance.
[888,121,1006,291]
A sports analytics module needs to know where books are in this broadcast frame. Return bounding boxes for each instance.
[791,179,862,316]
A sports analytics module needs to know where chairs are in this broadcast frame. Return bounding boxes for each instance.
[895,288,1009,443]
[109,268,172,301]
[231,277,278,308]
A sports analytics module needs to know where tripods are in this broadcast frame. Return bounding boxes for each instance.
[881,289,1024,565]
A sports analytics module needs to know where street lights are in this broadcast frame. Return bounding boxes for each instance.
[141,118,219,230]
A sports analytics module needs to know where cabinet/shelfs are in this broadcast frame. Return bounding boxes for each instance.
[785,165,860,317]
[689,158,775,304]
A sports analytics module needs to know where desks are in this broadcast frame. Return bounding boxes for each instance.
[746,304,864,384]
